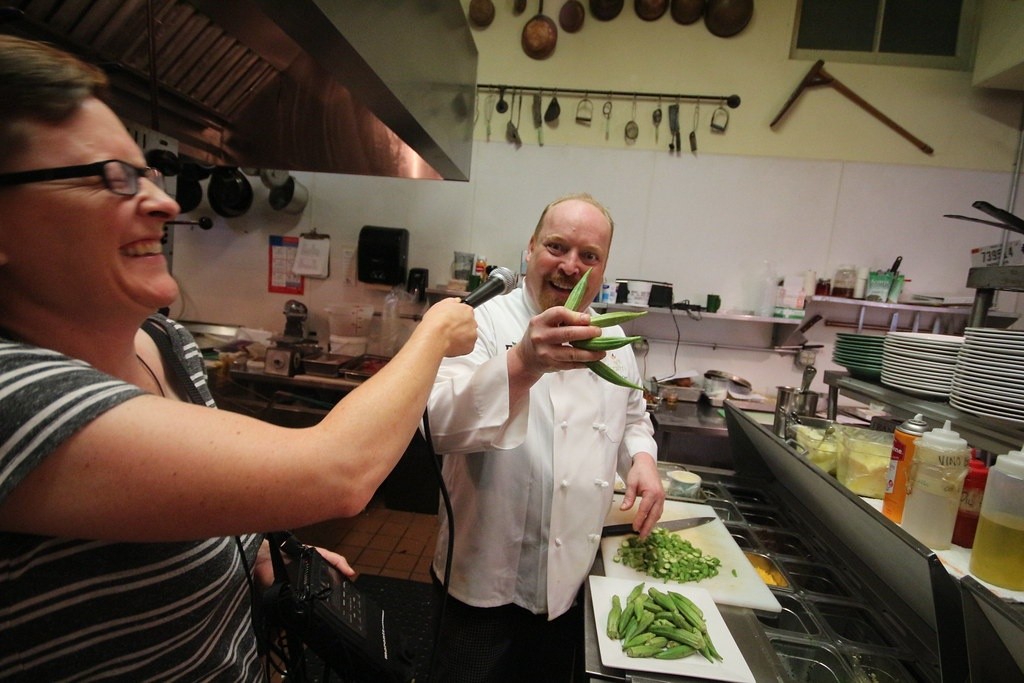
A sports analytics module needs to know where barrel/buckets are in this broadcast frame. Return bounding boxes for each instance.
[773,385,818,438]
[328,335,368,358]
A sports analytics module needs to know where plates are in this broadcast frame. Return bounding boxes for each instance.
[589,575,758,683]
[830,326,1024,429]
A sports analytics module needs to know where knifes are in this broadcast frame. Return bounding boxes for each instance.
[778,314,823,356]
[601,517,715,538]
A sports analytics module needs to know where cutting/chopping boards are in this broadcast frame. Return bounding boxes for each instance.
[601,494,783,612]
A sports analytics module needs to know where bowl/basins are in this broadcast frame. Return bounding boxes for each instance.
[324,304,374,337]
[666,471,702,498]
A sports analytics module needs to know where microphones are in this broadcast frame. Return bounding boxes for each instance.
[459,265,515,310]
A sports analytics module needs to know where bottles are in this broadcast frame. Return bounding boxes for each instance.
[882,413,930,522]
[902,419,968,549]
[969,446,1024,591]
[815,279,831,296]
[832,264,857,298]
[476,257,487,284]
[951,447,989,548]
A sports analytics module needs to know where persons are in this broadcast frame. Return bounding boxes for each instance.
[419,188,665,683]
[0,30,479,683]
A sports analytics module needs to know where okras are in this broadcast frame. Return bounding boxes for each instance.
[560,267,648,391]
[613,528,738,584]
[607,582,723,663]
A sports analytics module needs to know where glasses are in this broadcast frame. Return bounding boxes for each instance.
[0,159,165,196]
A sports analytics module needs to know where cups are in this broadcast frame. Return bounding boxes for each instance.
[454,251,475,280]
